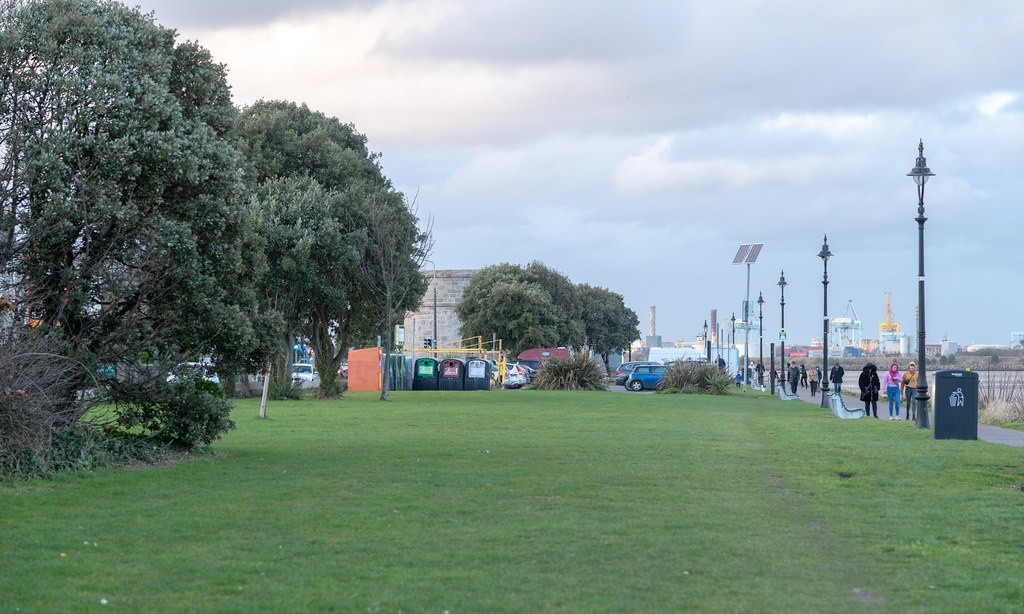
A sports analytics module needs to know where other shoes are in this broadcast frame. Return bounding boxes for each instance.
[912,416,916,420]
[874,416,879,419]
[906,415,909,419]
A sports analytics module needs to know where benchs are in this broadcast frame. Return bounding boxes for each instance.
[776,384,799,401]
[749,378,761,391]
[826,392,865,419]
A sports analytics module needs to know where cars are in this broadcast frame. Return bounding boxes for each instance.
[505,363,526,388]
[615,361,664,391]
[520,365,537,382]
[521,367,530,384]
[290,363,321,389]
[626,365,675,392]
[166,362,220,385]
[491,366,510,386]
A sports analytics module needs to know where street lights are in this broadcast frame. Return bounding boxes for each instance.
[907,139,934,428]
[756,291,766,388]
[703,320,708,347]
[731,313,736,345]
[776,269,789,394]
[818,233,833,407]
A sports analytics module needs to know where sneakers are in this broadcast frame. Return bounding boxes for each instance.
[895,416,901,420]
[890,416,894,420]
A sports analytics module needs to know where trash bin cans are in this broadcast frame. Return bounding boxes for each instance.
[465,359,490,391]
[440,359,464,391]
[932,370,978,441]
[413,358,438,390]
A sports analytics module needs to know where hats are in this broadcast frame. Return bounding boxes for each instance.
[834,363,839,366]
[791,361,796,364]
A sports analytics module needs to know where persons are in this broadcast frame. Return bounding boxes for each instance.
[747,362,765,376]
[900,362,918,420]
[735,372,742,387]
[715,354,726,369]
[494,356,504,384]
[830,362,844,394]
[859,362,880,418]
[787,361,807,395]
[809,366,822,396]
[882,364,902,420]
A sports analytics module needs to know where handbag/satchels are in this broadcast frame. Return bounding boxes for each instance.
[904,372,914,384]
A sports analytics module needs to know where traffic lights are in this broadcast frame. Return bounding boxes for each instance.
[428,339,432,346]
[424,339,427,348]
